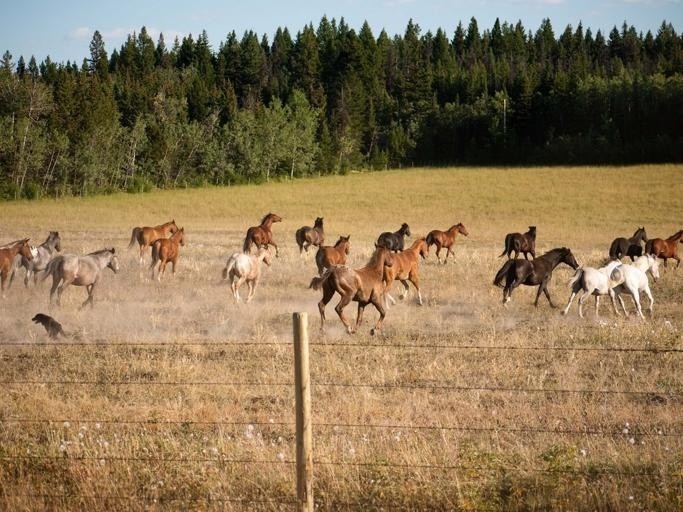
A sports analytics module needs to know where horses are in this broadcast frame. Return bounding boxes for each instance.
[39,247,119,311]
[244,211,283,259]
[502,226,537,260]
[609,226,650,263]
[0,237,34,301]
[493,245,580,309]
[425,222,470,266]
[17,231,62,290]
[559,258,626,320]
[615,253,662,326]
[308,241,394,337]
[382,234,429,311]
[294,216,325,258]
[377,222,410,253]
[646,230,682,271]
[146,227,186,281]
[221,242,272,305]
[316,234,350,277]
[127,218,180,267]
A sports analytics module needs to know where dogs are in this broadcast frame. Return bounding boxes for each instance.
[29,313,69,342]
